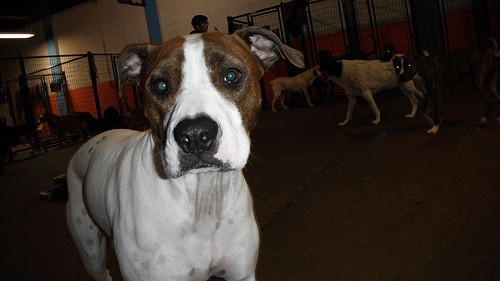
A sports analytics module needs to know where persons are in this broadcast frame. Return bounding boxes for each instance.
[189,15,209,34]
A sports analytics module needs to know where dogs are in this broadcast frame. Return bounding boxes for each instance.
[66,26,306,281]
[269,65,322,113]
[0,117,49,161]
[319,49,430,125]
[389,38,500,134]
[39,106,124,146]
